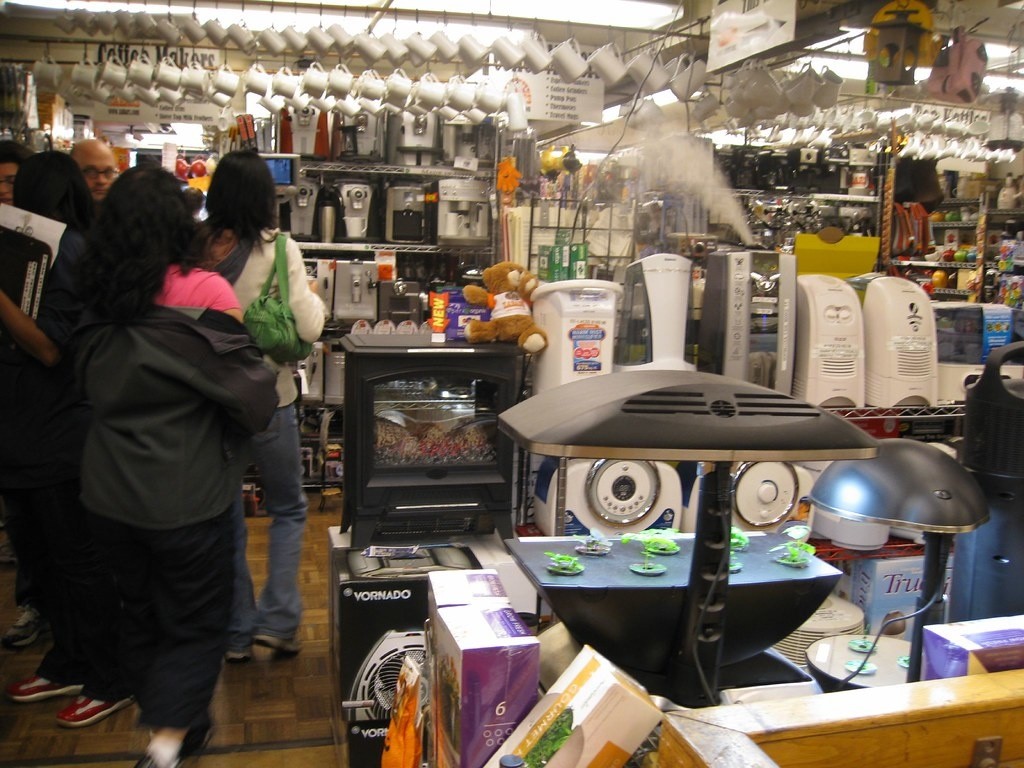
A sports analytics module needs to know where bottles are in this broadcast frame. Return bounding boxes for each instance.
[320,192,338,243]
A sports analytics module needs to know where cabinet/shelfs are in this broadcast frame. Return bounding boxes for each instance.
[277,159,496,508]
[883,195,1024,307]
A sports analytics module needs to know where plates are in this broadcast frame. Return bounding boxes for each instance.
[351,320,433,334]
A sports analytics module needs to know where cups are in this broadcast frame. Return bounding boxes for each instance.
[549,38,719,122]
[895,104,1016,164]
[727,59,844,122]
[852,173,869,188]
[258,27,553,71]
[55,9,257,55]
[32,56,240,106]
[246,63,528,132]
[345,217,368,238]
[759,105,895,148]
[447,213,464,235]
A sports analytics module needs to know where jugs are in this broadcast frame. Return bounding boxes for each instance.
[997,172,1022,210]
[24,129,53,154]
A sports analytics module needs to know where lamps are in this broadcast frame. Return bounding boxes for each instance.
[812,443,991,692]
[496,370,876,713]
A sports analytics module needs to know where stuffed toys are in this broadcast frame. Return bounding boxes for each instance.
[463,262,548,355]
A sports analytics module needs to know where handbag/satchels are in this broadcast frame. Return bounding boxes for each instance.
[243,234,313,361]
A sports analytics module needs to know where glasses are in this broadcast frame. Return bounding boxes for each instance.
[80,167,120,180]
[0,175,16,186]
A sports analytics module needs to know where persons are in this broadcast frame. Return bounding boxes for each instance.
[62,166,279,768]
[0,140,132,726]
[205,150,325,662]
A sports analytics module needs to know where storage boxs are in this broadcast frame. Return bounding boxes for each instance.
[980,302,1014,360]
[567,244,589,280]
[38,92,94,150]
[537,245,569,283]
[426,568,539,768]
[483,644,711,768]
[918,616,1024,683]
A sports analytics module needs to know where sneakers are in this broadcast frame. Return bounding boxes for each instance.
[7,675,84,701]
[4,605,45,646]
[56,691,134,728]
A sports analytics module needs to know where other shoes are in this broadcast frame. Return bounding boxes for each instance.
[226,645,251,663]
[133,711,213,768]
[253,632,305,655]
